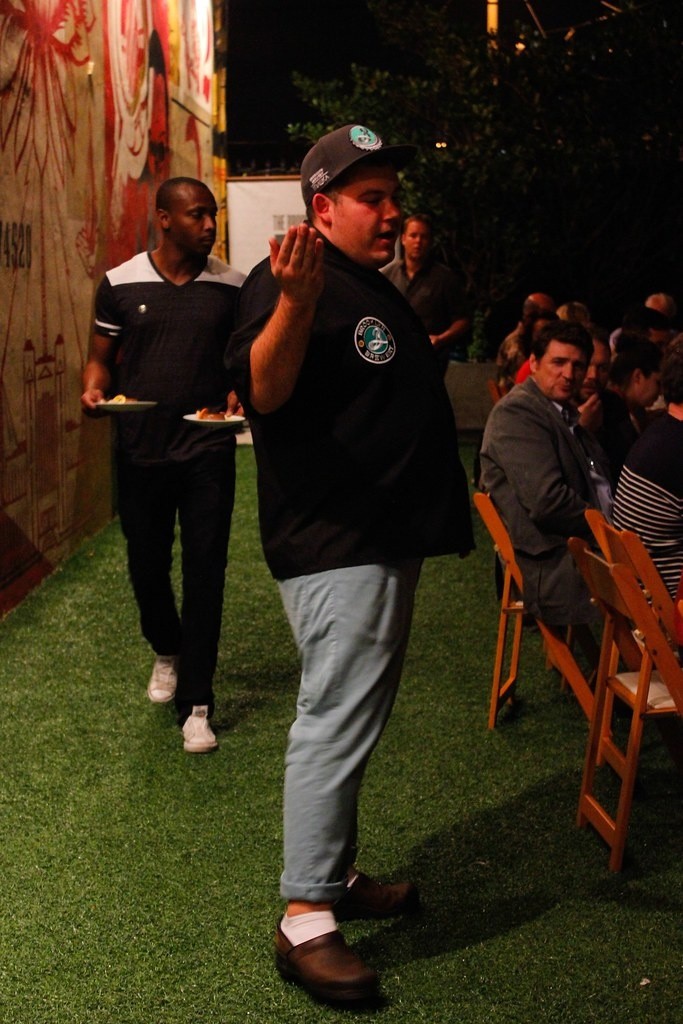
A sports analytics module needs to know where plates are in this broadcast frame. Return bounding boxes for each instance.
[96,401,158,412]
[183,414,245,428]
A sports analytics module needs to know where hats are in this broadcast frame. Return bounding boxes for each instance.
[301,124,419,209]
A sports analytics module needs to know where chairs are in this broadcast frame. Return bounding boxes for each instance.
[475,489,683,872]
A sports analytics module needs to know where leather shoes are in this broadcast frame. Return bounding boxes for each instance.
[333,871,420,922]
[275,912,380,1001]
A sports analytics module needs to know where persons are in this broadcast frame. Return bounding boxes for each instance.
[225,125,477,1009]
[80,175,250,753]
[374,213,470,379]
[480,320,603,628]
[489,292,683,647]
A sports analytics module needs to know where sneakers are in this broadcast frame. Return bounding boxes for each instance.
[147,654,178,702]
[183,706,217,752]
